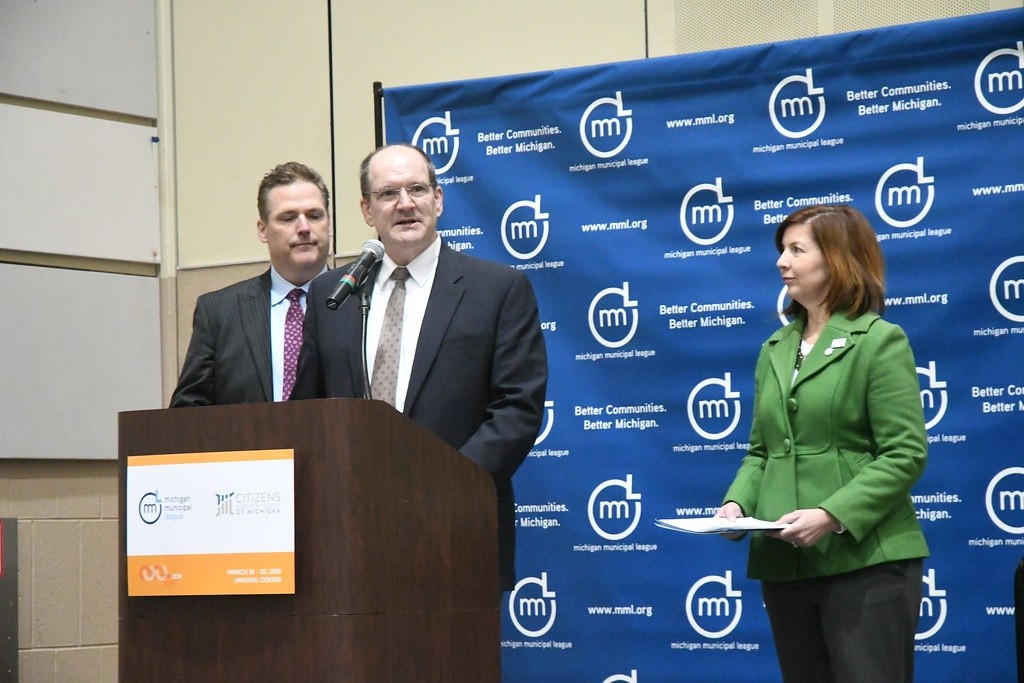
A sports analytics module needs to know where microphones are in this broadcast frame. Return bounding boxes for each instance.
[326,239,386,310]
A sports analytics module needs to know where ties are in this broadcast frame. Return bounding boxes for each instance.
[369,267,411,410]
[282,288,306,401]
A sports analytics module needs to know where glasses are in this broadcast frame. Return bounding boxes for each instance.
[363,181,440,202]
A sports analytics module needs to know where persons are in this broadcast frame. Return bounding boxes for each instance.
[709,206,930,683]
[286,144,550,602]
[168,160,333,407]
